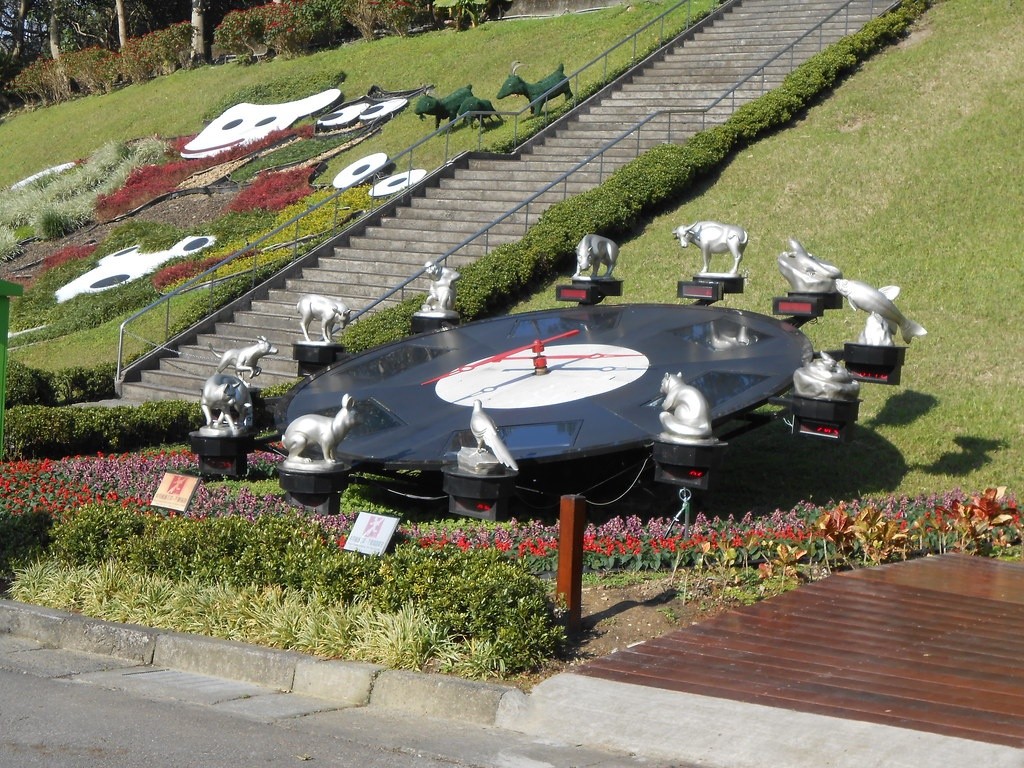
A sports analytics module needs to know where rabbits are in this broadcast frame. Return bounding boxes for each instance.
[281,393,364,464]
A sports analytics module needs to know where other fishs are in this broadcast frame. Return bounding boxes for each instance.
[835,278,928,345]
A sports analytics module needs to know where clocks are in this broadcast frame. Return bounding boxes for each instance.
[277,304,816,480]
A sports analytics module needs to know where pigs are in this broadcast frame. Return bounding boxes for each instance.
[572,233,620,278]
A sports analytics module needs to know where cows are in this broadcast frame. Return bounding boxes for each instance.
[672,220,749,275]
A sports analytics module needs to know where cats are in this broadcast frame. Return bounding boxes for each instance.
[200,371,253,437]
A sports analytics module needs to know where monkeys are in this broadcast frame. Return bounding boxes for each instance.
[421,259,461,312]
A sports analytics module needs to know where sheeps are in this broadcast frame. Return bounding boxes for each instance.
[496,61,574,118]
[296,293,352,343]
[458,96,503,132]
[414,82,475,138]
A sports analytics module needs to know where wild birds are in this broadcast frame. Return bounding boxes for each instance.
[469,400,520,471]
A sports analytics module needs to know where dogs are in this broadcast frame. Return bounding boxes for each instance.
[208,335,279,387]
